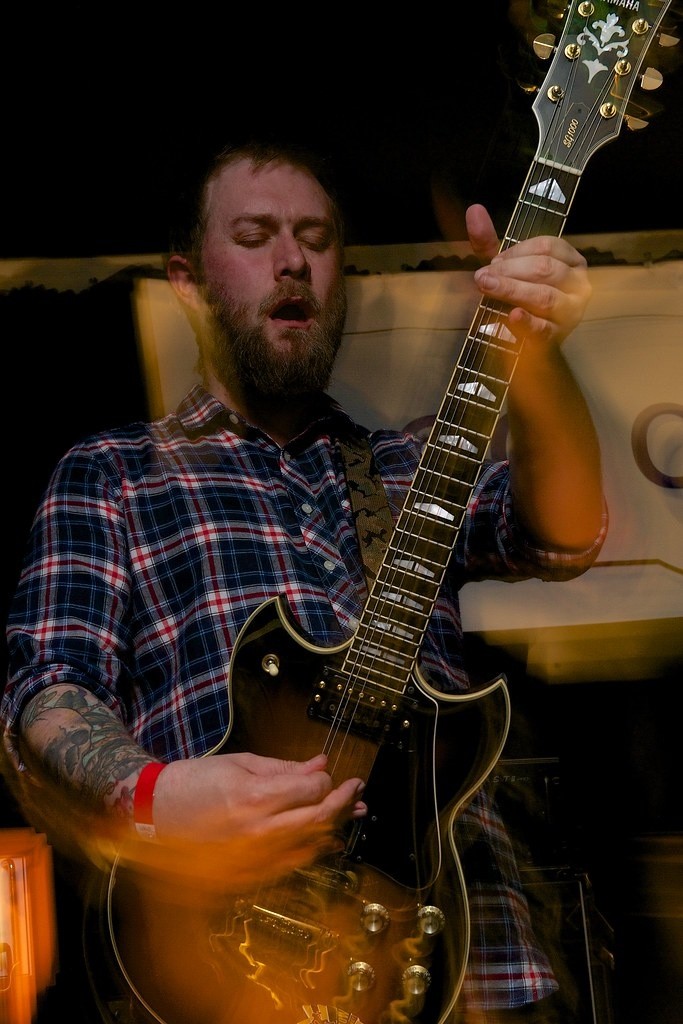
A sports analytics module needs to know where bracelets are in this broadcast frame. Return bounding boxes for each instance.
[133,762,167,844]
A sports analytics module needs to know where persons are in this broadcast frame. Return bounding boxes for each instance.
[0,133,608,1024]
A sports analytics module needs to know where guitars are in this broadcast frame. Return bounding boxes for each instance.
[107,3,680,1021]
